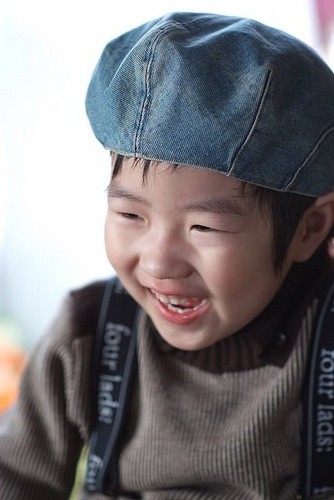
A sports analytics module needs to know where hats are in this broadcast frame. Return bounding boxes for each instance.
[84,11,334,199]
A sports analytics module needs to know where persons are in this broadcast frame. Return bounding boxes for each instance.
[0,10,333,500]
